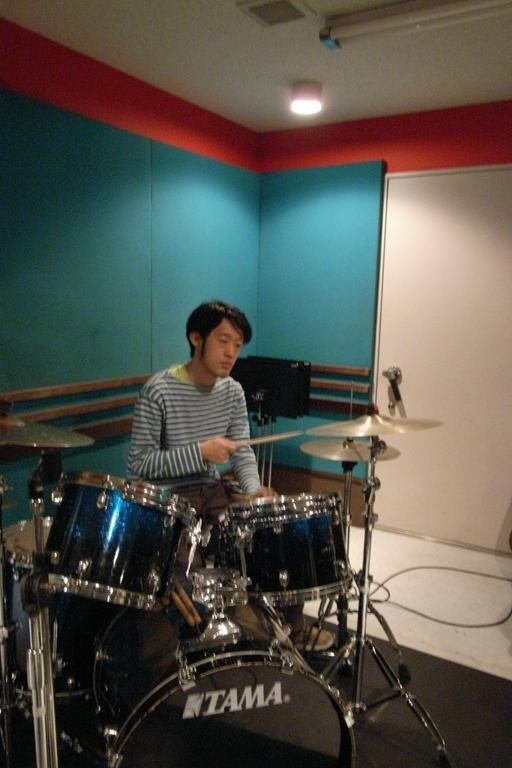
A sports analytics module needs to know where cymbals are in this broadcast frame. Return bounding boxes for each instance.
[299,439,401,462]
[304,415,447,437]
[1,417,94,449]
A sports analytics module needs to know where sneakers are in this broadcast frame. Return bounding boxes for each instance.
[290,624,336,652]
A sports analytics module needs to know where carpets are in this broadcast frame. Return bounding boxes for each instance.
[2,607,512,768]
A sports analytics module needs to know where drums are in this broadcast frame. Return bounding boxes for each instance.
[92,567,356,767]
[1,517,116,697]
[216,491,354,605]
[33,471,213,612]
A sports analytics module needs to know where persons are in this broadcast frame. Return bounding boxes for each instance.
[126,299,336,652]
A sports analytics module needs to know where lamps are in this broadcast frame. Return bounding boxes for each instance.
[317,1,512,53]
[284,80,326,119]
